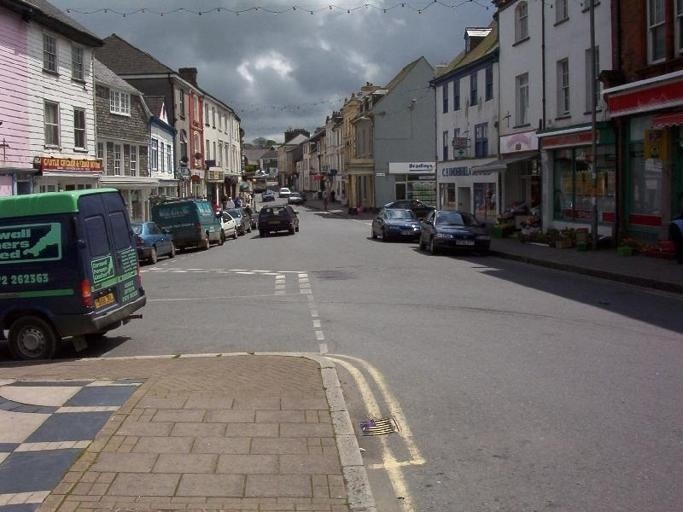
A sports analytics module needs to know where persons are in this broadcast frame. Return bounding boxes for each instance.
[323,192,329,211]
[212,196,242,213]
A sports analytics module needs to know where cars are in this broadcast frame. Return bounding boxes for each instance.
[372,209,421,242]
[132,222,175,264]
[419,210,491,257]
[383,199,436,217]
[257,204,299,236]
[279,187,302,204]
[262,190,275,202]
[215,205,258,239]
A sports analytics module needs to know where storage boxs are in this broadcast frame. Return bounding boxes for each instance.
[556,240,572,249]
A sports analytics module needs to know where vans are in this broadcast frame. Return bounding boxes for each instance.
[0,188,146,361]
[152,200,224,249]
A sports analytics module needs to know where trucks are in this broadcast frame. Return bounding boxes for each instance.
[253,178,267,193]
